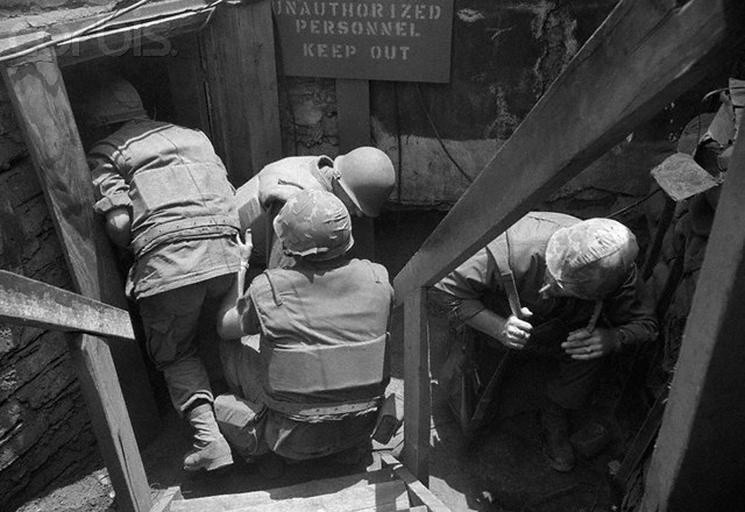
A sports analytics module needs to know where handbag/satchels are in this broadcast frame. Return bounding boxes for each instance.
[211,392,268,461]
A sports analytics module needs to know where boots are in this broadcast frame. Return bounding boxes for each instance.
[179,400,235,474]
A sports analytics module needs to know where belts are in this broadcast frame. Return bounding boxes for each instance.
[132,213,242,255]
[264,392,386,418]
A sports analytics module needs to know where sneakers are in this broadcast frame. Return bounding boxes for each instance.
[536,399,578,473]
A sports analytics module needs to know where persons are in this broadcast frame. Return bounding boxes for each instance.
[431,210,661,474]
[234,145,396,266]
[78,74,247,472]
[210,190,397,466]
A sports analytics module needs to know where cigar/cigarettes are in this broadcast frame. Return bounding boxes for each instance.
[538,284,550,293]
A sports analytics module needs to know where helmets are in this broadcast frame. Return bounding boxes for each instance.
[75,73,150,131]
[331,145,397,219]
[545,216,642,304]
[273,188,354,262]
